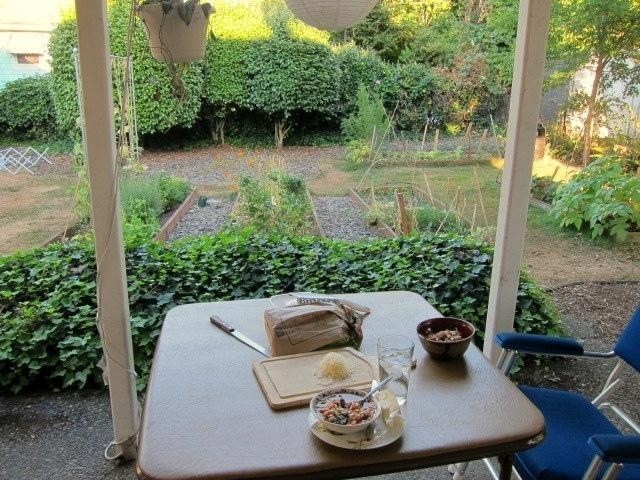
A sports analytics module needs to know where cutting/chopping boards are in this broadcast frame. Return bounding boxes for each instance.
[252,347,418,410]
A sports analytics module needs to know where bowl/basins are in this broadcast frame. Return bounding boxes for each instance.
[309,388,382,434]
[416,316,476,358]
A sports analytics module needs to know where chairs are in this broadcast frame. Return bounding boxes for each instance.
[453,301,640,480]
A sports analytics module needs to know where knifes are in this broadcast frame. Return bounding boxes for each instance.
[209,314,272,357]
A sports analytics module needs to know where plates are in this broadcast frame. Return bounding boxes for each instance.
[308,392,408,451]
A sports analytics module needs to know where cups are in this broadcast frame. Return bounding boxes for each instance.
[378,335,415,406]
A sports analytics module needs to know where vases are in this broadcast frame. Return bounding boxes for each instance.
[134,3,211,63]
